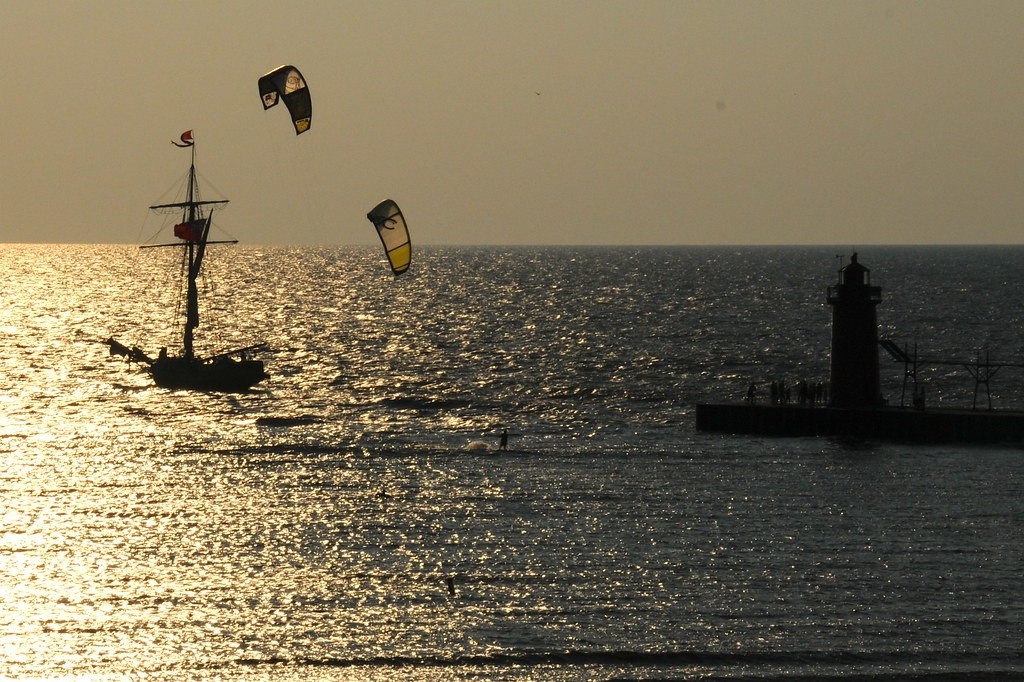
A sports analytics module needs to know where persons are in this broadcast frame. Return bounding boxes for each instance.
[499,431,509,449]
[747,380,823,405]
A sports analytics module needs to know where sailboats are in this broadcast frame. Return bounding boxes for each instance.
[106,129,266,393]
